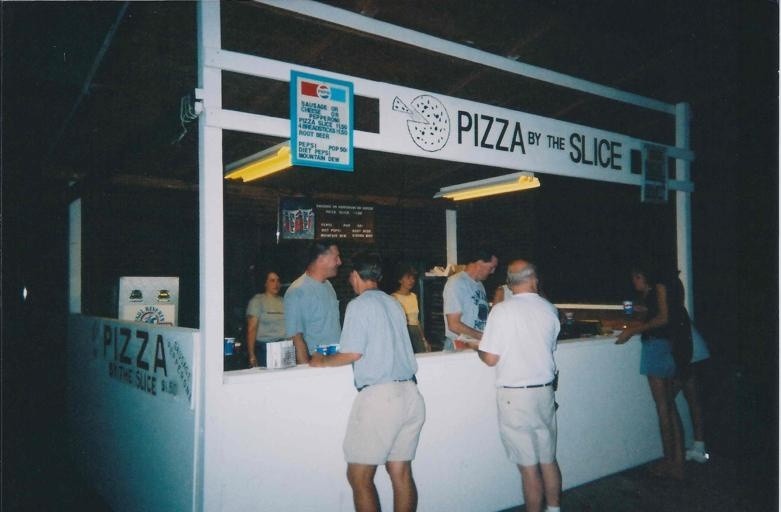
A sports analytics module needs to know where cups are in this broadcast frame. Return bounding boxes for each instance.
[282,208,311,233]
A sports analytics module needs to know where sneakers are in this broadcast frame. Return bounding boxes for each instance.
[686,448,711,463]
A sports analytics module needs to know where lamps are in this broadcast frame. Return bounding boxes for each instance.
[224,139,292,182]
[432,171,541,201]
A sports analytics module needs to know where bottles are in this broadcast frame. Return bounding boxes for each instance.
[624,302,632,318]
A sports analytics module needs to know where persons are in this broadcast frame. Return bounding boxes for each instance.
[309,250,426,512]
[614,255,710,478]
[477,261,562,512]
[284,240,342,364]
[246,272,283,366]
[391,265,432,354]
[442,240,499,350]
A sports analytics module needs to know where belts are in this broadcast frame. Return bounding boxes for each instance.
[504,383,552,388]
[357,378,417,392]
